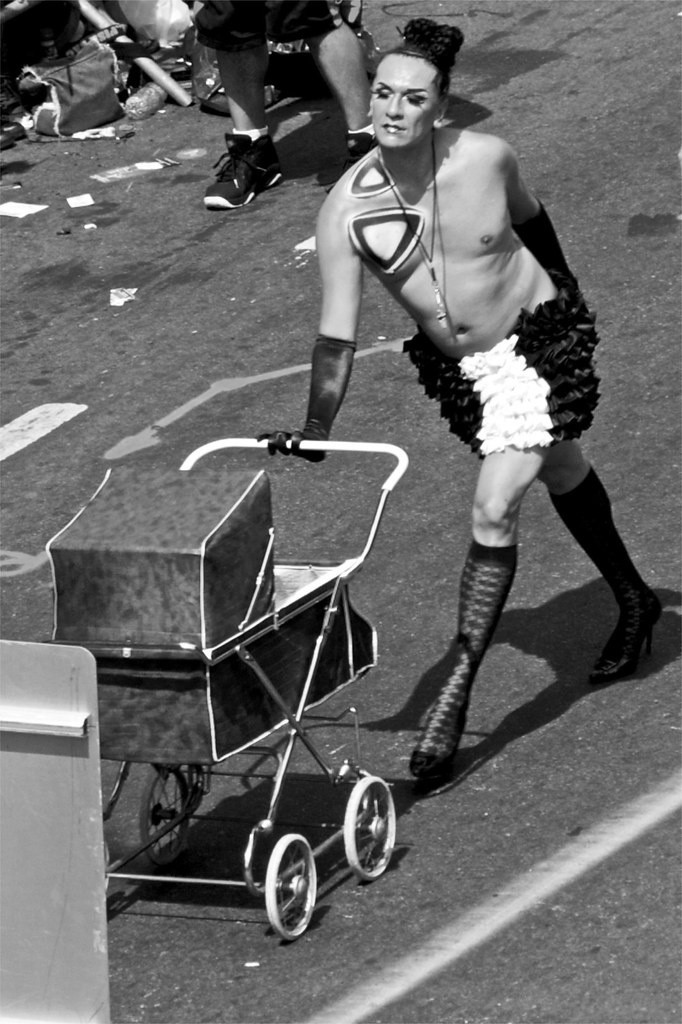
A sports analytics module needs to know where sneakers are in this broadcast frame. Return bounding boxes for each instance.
[203,131,284,210]
[324,133,375,194]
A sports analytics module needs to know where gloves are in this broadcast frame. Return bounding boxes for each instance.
[509,198,589,318]
[256,332,357,463]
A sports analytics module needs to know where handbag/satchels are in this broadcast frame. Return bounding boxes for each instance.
[15,40,125,140]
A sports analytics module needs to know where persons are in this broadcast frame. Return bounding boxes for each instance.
[256,18,664,776]
[182,0,378,210]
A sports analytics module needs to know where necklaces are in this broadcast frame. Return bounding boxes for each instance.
[377,126,448,328]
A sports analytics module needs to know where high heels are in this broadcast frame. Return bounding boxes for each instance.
[588,590,662,682]
[409,695,485,778]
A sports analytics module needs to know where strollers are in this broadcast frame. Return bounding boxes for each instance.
[49,436,410,942]
